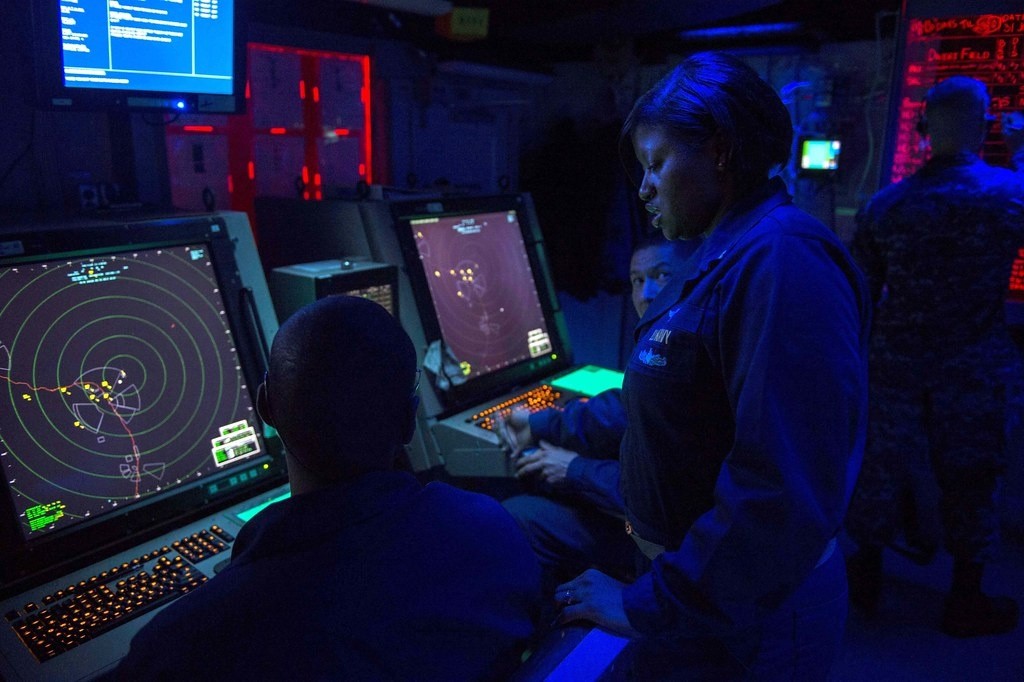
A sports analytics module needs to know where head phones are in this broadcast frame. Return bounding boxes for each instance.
[914,76,995,136]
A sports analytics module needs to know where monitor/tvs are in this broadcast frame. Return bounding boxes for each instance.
[33,0,246,115]
[795,136,845,180]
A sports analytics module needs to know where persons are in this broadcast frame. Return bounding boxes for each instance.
[88,295,557,682]
[498,51,868,682]
[856,74,1023,636]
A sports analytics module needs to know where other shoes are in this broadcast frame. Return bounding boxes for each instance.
[937,594,1019,637]
[849,571,887,615]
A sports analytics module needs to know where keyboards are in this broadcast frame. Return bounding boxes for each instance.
[465,385,592,436]
[6,525,235,663]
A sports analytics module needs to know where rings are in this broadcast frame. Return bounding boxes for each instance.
[562,589,575,606]
